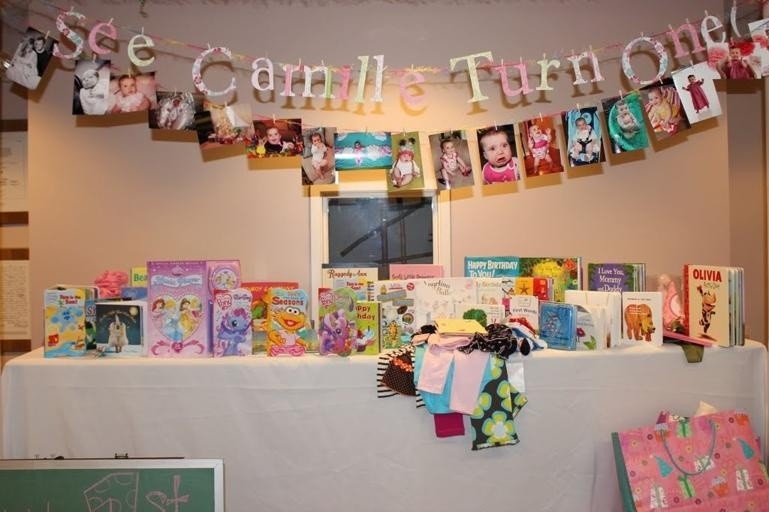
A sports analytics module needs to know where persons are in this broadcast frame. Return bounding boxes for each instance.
[310,132,329,181]
[353,139,365,167]
[437,139,472,190]
[526,125,556,174]
[6,38,42,92]
[615,100,641,141]
[34,36,50,77]
[715,28,769,81]
[78,70,107,114]
[478,128,520,185]
[681,74,711,114]
[571,115,601,161]
[390,150,420,188]
[644,87,681,136]
[110,75,152,117]
[163,98,183,129]
[261,126,296,153]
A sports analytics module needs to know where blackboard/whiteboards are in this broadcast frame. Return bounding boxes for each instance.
[0,457,226,512]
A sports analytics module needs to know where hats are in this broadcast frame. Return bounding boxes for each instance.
[398,137,415,156]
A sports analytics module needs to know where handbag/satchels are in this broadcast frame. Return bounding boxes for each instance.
[611,408,769,512]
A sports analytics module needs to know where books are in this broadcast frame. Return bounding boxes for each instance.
[540,300,576,350]
[43,260,312,353]
[589,263,647,337]
[564,289,621,348]
[684,264,744,346]
[623,291,663,346]
[318,256,583,353]
[572,305,607,351]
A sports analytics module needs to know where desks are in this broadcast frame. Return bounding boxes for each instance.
[0,339,769,510]
